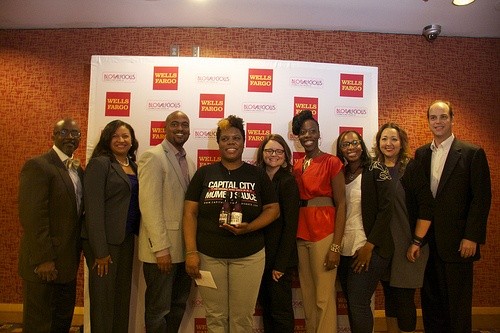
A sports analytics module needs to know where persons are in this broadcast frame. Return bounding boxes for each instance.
[336,130,393,333]
[372,122,434,333]
[291,108,347,333]
[80,120,141,333]
[136,111,197,333]
[414,101,492,333]
[17,119,84,333]
[253,134,300,333]
[183,115,281,333]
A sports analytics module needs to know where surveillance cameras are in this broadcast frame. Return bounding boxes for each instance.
[423,24,440,42]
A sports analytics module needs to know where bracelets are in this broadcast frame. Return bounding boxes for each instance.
[411,234,423,246]
[330,244,344,254]
[184,251,199,255]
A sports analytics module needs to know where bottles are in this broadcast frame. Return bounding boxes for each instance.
[230,193,243,227]
[219,192,230,228]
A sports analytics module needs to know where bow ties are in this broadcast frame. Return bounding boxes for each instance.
[66,159,81,169]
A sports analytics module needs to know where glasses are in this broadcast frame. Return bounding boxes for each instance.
[55,128,81,139]
[264,148,286,156]
[340,139,360,148]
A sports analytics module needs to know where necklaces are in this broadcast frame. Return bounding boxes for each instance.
[346,164,359,180]
[112,152,129,167]
[303,150,321,170]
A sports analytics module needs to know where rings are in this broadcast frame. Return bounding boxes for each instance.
[98,265,101,268]
[361,264,364,267]
[335,265,336,267]
